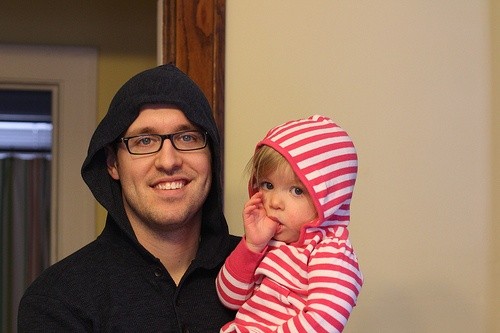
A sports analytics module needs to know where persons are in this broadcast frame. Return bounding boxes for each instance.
[17,63,242,333]
[215,115,364,333]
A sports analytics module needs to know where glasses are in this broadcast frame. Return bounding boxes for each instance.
[116,129,208,155]
